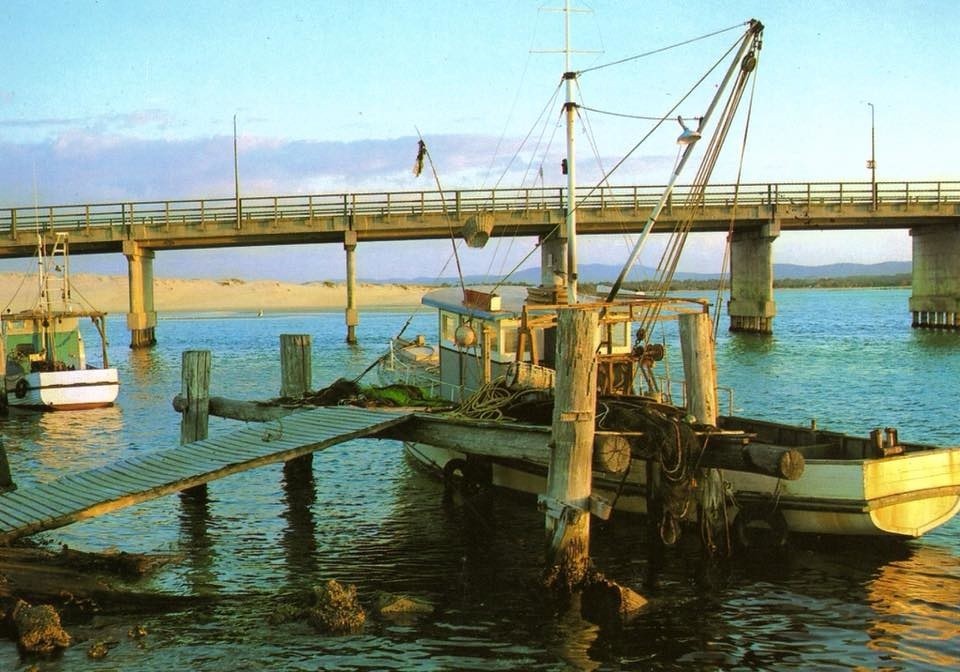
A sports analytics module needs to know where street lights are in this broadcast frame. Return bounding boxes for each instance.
[232,115,243,230]
[867,101,878,210]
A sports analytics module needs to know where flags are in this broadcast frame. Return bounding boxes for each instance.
[412,139,427,177]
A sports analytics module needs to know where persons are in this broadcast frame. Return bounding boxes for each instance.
[55,362,75,371]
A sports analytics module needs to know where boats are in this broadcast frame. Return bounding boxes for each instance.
[368,4,960,542]
[0,204,123,408]
